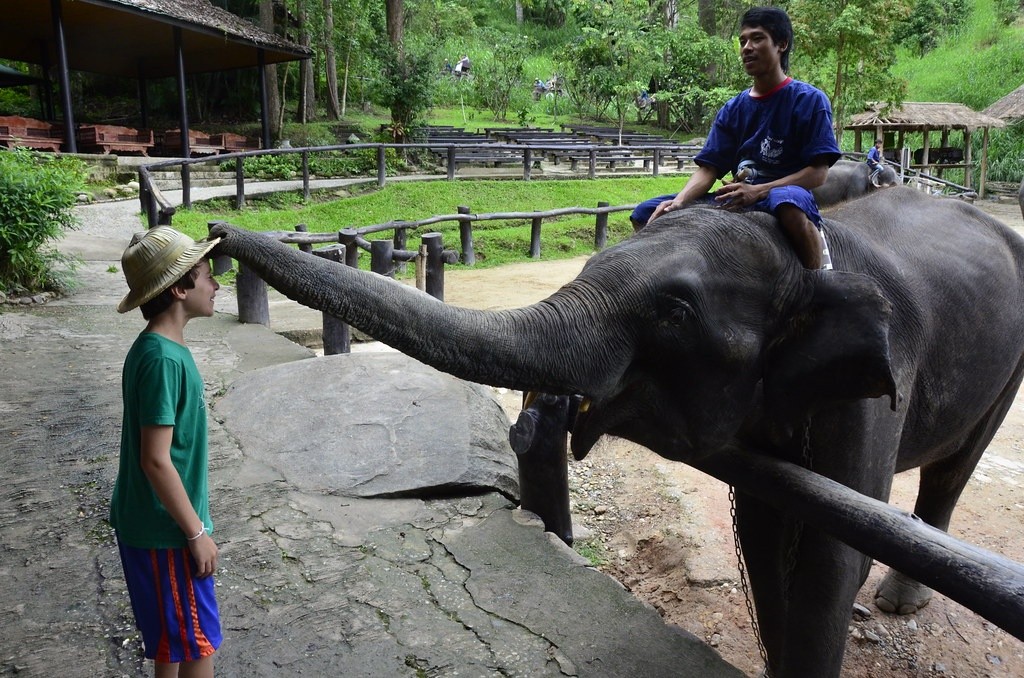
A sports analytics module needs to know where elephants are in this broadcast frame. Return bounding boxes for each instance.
[202,183,1024,678]
[811,160,902,210]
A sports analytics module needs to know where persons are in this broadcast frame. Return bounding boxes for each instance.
[444,54,471,72]
[640,85,649,109]
[110,226,224,678]
[866,139,884,185]
[534,71,563,93]
[630,8,841,270]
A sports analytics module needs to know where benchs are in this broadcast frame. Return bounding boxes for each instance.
[78,125,154,157]
[164,128,225,156]
[217,132,261,152]
[0,115,64,153]
[380,123,704,169]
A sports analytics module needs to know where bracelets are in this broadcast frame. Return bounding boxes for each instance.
[187,521,205,540]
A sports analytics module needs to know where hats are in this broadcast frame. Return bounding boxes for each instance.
[116,225,221,313]
